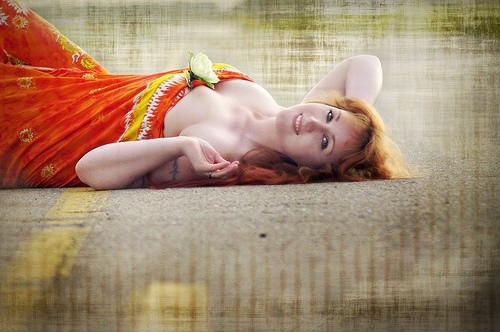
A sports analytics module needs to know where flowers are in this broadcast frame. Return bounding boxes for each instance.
[182,50,221,90]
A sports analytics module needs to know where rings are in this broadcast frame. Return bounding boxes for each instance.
[209,171,212,178]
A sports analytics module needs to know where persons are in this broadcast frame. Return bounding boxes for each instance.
[0,0,409,191]
